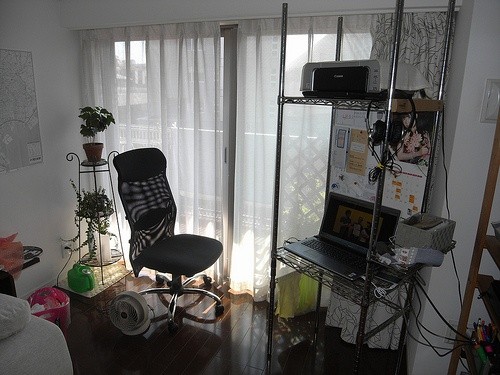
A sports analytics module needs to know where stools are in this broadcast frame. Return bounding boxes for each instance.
[0,293,73,375]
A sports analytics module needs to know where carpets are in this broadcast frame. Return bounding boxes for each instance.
[57,264,132,298]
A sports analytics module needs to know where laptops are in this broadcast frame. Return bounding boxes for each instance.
[284,192,402,279]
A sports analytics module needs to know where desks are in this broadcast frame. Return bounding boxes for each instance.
[0,246,43,298]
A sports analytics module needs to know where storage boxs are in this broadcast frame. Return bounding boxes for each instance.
[395,213,456,253]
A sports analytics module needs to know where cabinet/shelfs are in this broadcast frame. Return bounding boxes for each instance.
[246,0,500,375]
[57,151,127,288]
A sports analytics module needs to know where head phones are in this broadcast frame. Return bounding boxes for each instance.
[365,89,416,146]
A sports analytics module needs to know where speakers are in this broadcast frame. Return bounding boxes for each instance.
[300,59,381,99]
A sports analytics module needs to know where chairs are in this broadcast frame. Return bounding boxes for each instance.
[113,148,225,330]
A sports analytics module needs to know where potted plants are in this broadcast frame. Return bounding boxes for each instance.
[59,179,119,264]
[78,106,115,162]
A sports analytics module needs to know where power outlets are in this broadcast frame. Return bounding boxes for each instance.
[61,241,71,258]
